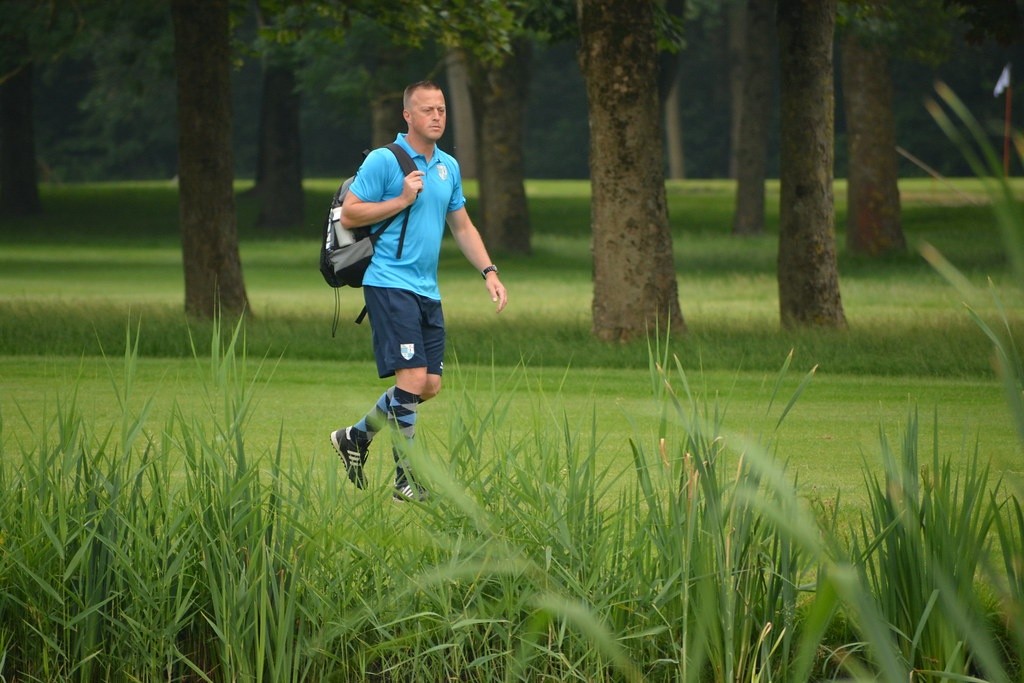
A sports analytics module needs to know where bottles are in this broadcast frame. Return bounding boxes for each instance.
[332,207,356,247]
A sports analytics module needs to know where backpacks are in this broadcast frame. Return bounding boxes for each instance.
[319,144,419,288]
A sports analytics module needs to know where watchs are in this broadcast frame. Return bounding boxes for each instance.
[481,264,498,280]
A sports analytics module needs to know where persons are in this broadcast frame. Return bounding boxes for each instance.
[329,78,508,504]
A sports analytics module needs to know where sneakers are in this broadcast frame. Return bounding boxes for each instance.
[393,480,438,505]
[330,426,369,491]
